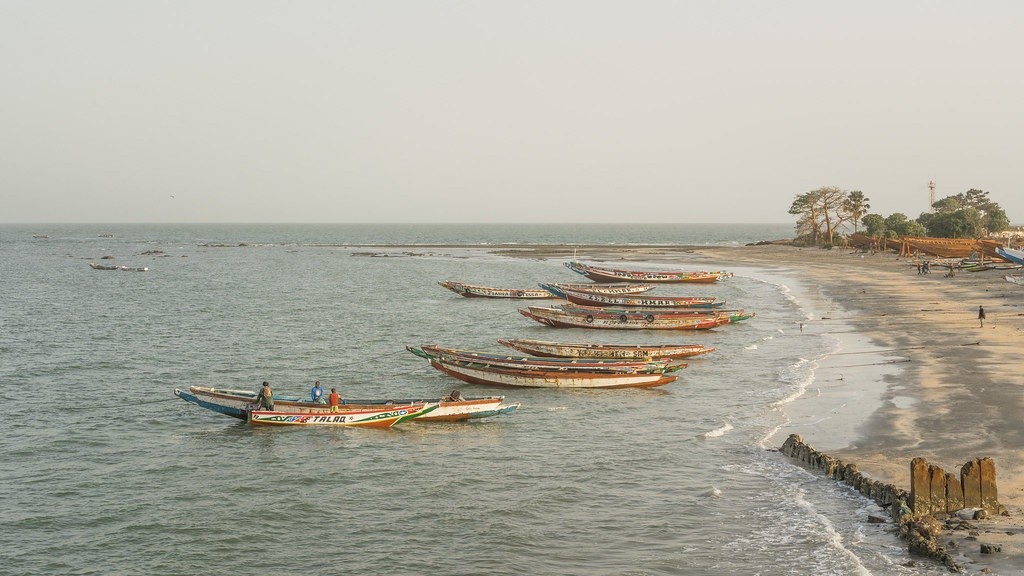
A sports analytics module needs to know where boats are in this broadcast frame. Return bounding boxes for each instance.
[539,281,726,309]
[175,385,521,421]
[244,400,442,429]
[439,279,658,298]
[517,305,756,330]
[563,259,734,281]
[497,339,717,358]
[407,343,689,389]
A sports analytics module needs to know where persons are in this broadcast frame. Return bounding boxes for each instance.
[915,253,963,278]
[328,388,340,413]
[311,381,327,405]
[251,382,275,412]
[977,305,986,329]
